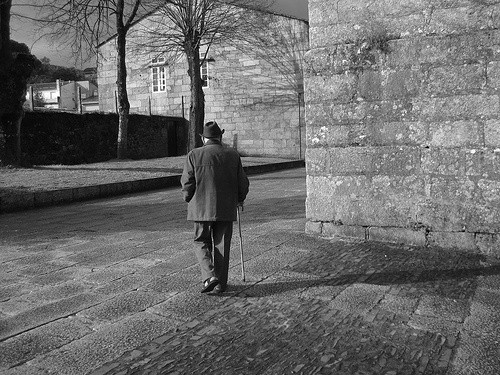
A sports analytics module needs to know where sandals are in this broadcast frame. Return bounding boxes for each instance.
[200,277,227,293]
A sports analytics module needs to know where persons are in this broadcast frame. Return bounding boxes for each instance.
[180,121,250,296]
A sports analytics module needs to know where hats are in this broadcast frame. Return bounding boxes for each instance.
[199,120,225,138]
